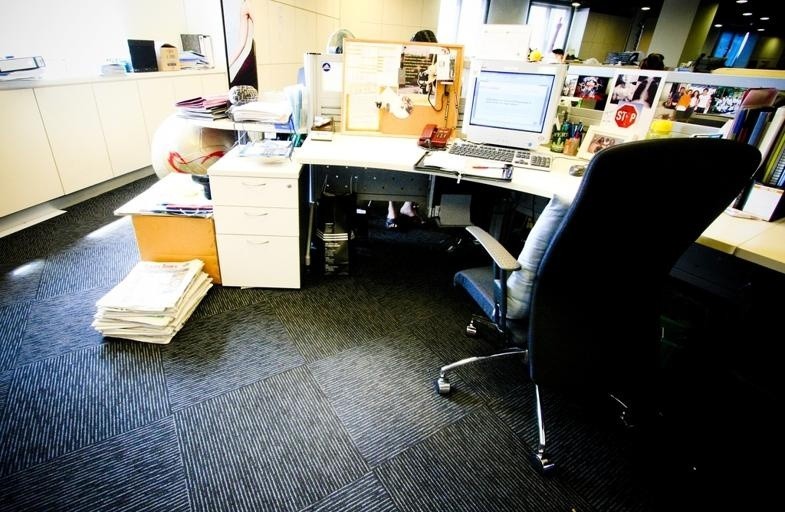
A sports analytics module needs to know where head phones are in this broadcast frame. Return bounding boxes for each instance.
[327,28,356,55]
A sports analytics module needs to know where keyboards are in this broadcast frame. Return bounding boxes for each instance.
[446,140,553,172]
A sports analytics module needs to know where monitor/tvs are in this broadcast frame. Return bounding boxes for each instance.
[471,24,532,62]
[462,59,567,152]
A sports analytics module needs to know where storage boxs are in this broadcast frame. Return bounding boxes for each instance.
[131,214,221,287]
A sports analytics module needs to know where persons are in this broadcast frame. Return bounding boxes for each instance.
[574,75,742,122]
[386,30,437,229]
[434,47,453,91]
[640,52,663,69]
[551,48,564,64]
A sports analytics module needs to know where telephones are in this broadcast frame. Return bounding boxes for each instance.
[418,124,452,148]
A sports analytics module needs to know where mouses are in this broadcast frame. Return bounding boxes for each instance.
[569,164,587,177]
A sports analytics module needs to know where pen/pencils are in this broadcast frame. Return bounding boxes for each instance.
[571,121,584,139]
[311,139,331,141]
[472,167,510,170]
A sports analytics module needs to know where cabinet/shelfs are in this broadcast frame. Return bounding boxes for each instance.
[207,141,314,291]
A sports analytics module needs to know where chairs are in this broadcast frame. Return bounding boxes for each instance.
[435,137,761,473]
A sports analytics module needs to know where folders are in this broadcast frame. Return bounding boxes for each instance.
[180,34,204,54]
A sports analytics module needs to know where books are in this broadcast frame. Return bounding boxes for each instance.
[727,85,785,189]
[415,150,513,184]
[240,138,295,164]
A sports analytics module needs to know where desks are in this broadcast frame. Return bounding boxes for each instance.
[299,130,785,278]
[181,113,295,149]
